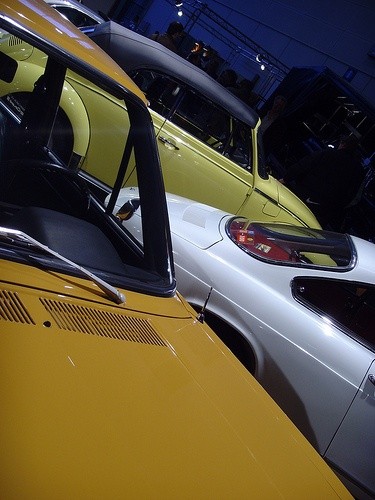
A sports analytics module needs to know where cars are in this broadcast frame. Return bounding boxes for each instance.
[42,0,105,31]
[0,20,339,271]
[106,186,375,500]
[0,0,357,500]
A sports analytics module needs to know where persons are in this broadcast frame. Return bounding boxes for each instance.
[143,23,366,213]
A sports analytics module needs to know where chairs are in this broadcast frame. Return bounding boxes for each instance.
[147,76,167,98]
